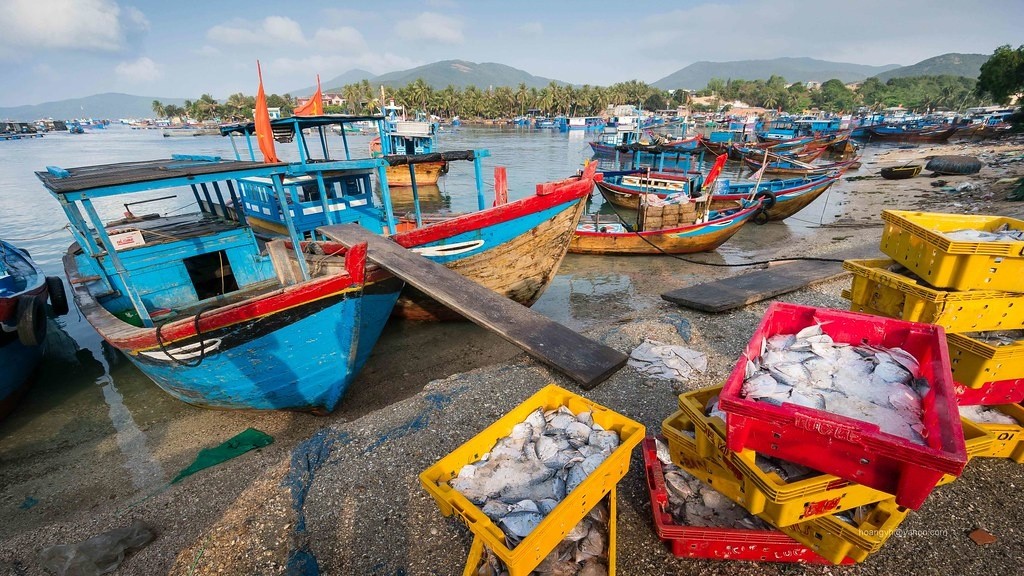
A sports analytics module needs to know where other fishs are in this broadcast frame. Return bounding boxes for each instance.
[442,401,622,576]
[649,220,1024,538]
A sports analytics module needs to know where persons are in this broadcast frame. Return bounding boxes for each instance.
[986,118,989,122]
[943,117,948,124]
[878,115,884,124]
[861,113,866,124]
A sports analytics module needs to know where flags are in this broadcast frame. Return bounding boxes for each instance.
[293,87,322,117]
[703,154,727,190]
[255,80,278,164]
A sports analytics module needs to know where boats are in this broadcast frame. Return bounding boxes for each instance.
[371,85,445,188]
[487,100,1024,258]
[0,104,462,141]
[195,110,599,324]
[35,111,424,418]
[0,241,64,353]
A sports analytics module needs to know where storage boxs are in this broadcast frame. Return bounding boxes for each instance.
[106,228,145,251]
[417,195,1024,576]
